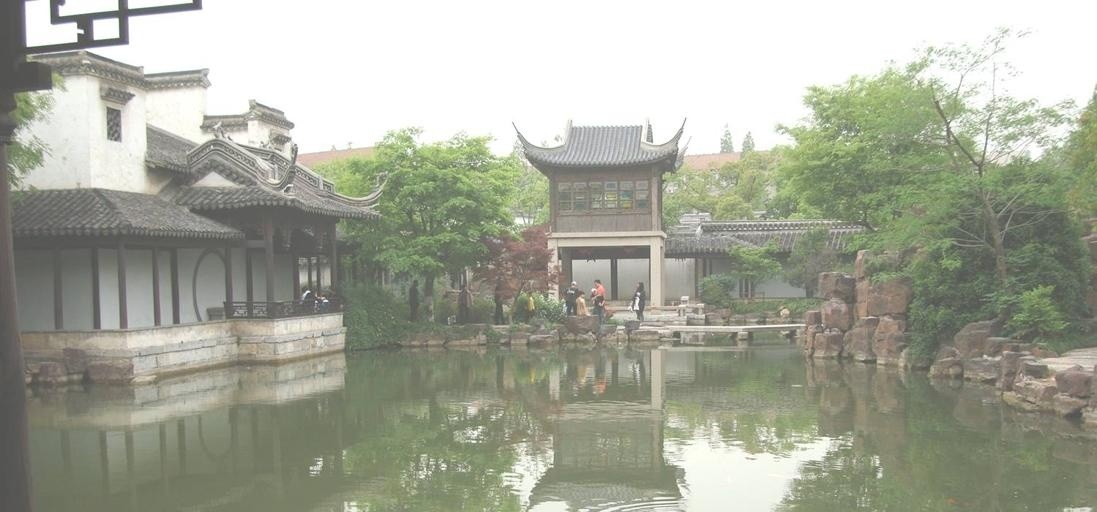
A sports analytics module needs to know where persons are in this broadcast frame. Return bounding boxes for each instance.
[495,286,505,324]
[299,287,339,314]
[563,279,606,325]
[458,284,472,324]
[631,282,646,320]
[528,292,534,318]
[409,279,420,322]
